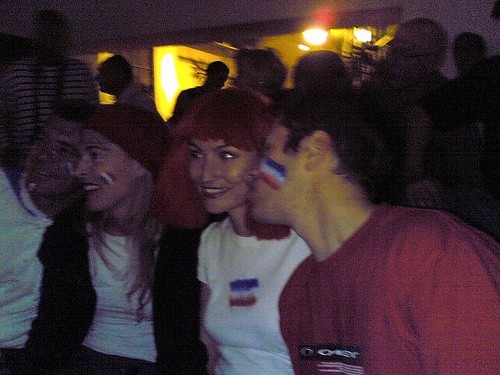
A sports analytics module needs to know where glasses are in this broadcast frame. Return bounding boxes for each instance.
[386,42,434,62]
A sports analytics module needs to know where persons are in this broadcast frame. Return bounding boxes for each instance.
[246,95,499,375]
[0,105,94,375]
[150,87,312,375]
[24,105,210,375]
[0,0,500,246]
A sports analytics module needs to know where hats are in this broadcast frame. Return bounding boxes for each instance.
[80,103,170,177]
[171,87,274,153]
